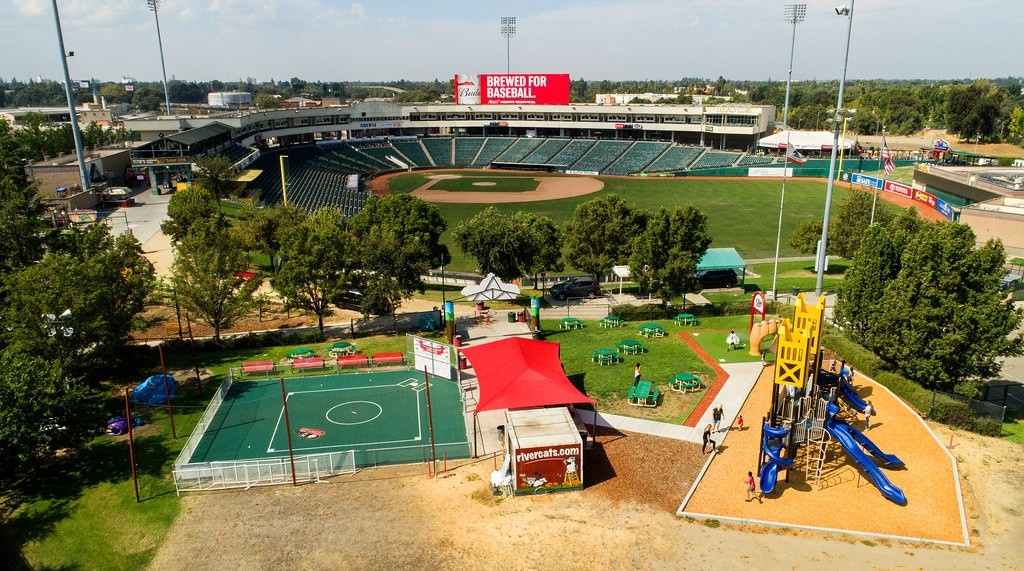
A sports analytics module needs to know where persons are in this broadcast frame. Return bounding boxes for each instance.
[830,360,837,372]
[634,363,641,386]
[726,330,740,351]
[847,407,858,419]
[761,349,766,366]
[745,471,761,502]
[738,415,743,430]
[702,424,719,454]
[863,399,872,429]
[847,367,854,384]
[711,404,725,433]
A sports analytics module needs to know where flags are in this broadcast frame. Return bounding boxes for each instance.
[881,139,895,177]
[787,143,804,167]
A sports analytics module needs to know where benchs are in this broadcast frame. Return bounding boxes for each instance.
[291,357,325,371]
[598,320,624,328]
[559,320,583,330]
[328,344,357,358]
[286,349,315,362]
[674,317,697,327]
[592,342,644,366]
[639,324,666,339]
[336,354,369,369]
[241,360,276,377]
[652,386,659,405]
[372,352,403,367]
[627,385,635,405]
[667,376,702,393]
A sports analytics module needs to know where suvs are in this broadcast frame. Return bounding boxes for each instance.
[550,275,601,301]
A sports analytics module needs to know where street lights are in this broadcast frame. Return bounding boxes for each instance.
[783,3,808,129]
[41,308,75,371]
[145,0,171,116]
[814,0,856,298]
[501,16,516,73]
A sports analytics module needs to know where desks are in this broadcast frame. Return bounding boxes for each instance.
[604,316,619,328]
[597,348,615,366]
[291,348,309,355]
[564,318,578,330]
[634,380,652,407]
[621,340,638,355]
[678,314,694,327]
[674,373,695,391]
[644,323,660,336]
[333,341,350,349]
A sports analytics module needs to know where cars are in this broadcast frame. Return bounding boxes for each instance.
[688,269,739,290]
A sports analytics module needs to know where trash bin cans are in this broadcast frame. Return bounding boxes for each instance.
[517,312,525,322]
[453,335,462,347]
[477,301,484,310]
[508,312,515,322]
[792,286,800,296]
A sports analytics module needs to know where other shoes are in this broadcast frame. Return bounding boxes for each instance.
[717,430,720,433]
[703,453,707,456]
[758,497,761,502]
[713,450,719,452]
[745,499,751,502]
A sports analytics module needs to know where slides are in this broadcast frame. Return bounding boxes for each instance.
[842,382,874,412]
[837,419,904,465]
[827,423,906,504]
[759,424,795,495]
